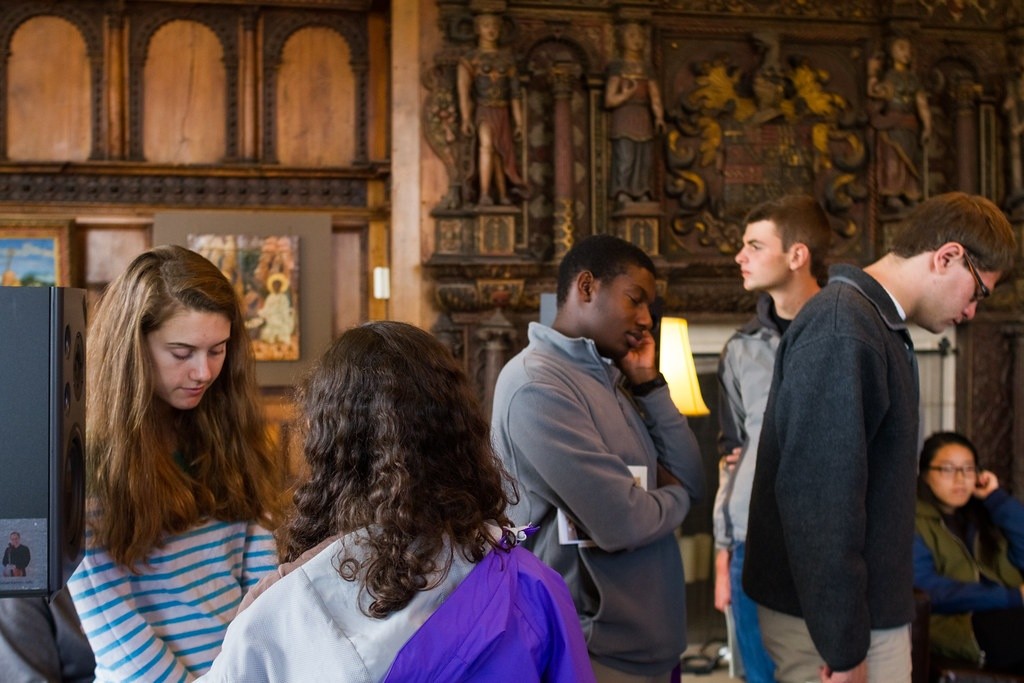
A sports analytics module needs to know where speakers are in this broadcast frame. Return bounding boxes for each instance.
[0,286,88,605]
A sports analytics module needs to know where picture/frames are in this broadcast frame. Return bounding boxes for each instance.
[0,212,77,287]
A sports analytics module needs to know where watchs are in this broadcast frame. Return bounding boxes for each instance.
[632,372,667,397]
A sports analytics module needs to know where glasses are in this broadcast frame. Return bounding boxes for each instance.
[930,464,978,479]
[963,255,991,304]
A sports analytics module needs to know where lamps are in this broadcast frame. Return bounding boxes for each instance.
[658,314,710,417]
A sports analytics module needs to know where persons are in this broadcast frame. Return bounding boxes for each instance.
[741,192,1018,683]
[458,11,523,205]
[3,532,30,576]
[0,584,97,683]
[718,192,834,683]
[607,22,667,201]
[65,243,280,683]
[867,36,931,210]
[188,320,597,683]
[489,234,704,681]
[911,433,1024,676]
